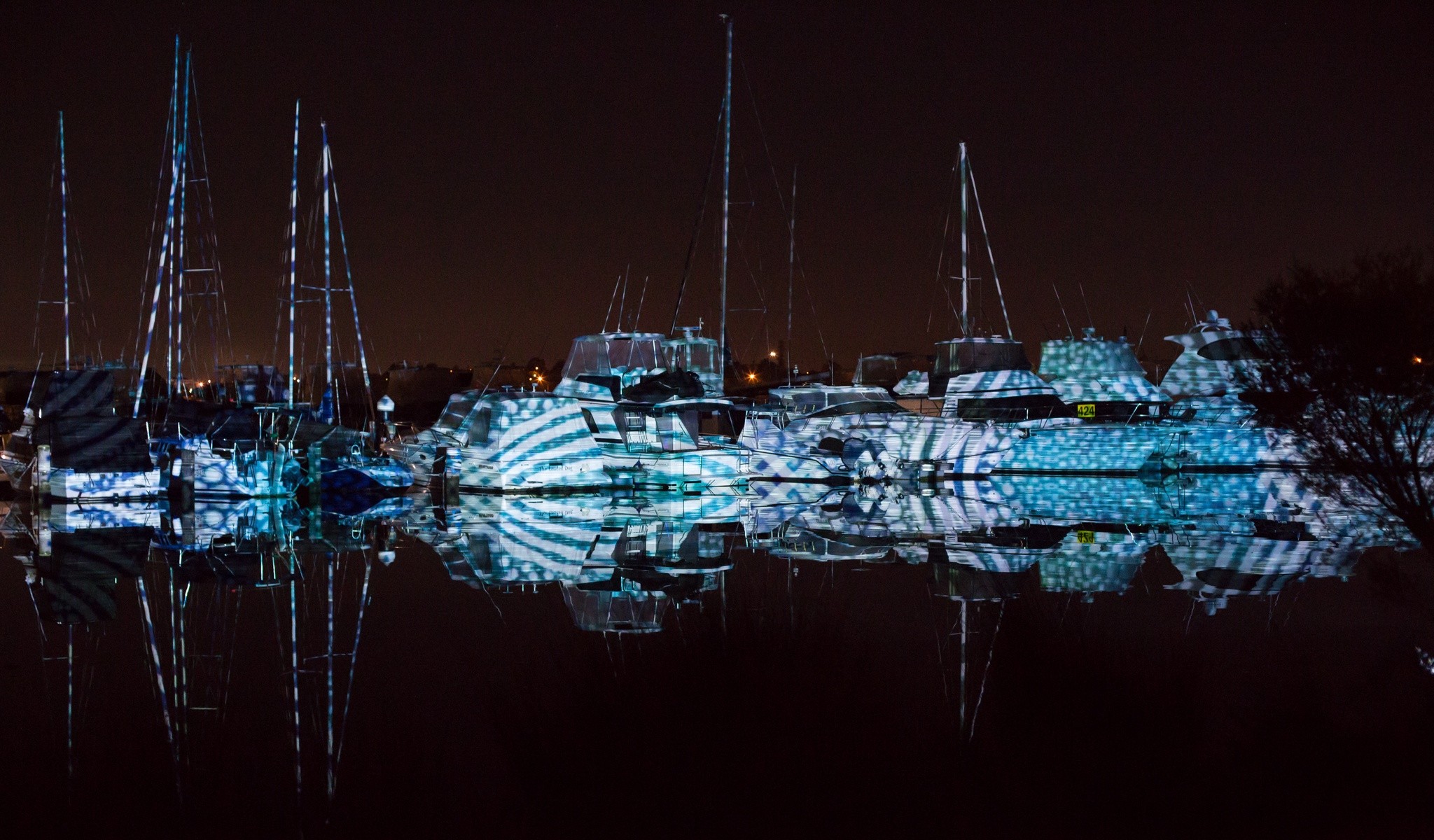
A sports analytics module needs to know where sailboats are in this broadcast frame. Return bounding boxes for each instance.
[0,9,1434,503]
[0,472,1434,802]
